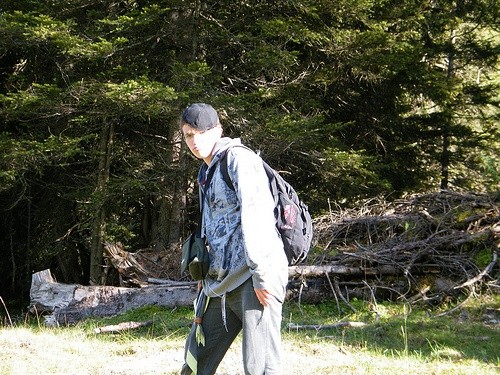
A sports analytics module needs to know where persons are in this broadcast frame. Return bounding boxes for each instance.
[180,102,289,375]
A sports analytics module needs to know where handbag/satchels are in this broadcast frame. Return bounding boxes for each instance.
[180,233,209,280]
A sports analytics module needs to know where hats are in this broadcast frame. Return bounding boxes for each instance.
[184,102,217,130]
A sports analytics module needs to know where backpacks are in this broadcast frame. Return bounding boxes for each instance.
[219,143,313,266]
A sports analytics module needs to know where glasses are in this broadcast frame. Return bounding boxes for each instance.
[183,125,216,141]
